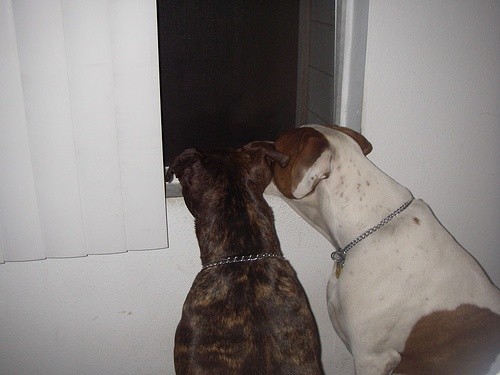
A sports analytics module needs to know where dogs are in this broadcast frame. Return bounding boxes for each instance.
[164,140,327,375]
[273,123,500,375]
